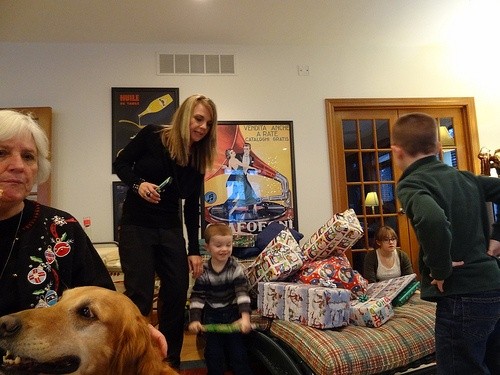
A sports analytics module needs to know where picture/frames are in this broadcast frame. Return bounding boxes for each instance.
[200,120,299,246]
[111,85,180,242]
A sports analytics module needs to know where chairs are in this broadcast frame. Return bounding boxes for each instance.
[250,298,438,375]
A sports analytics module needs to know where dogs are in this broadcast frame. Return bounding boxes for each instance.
[0,284,181,375]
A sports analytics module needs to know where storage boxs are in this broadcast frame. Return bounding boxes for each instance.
[241,207,421,330]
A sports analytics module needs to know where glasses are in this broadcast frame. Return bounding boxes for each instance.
[384,236,396,241]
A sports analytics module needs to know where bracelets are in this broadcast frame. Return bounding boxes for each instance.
[132,179,144,192]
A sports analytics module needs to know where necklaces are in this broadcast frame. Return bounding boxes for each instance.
[0,206,24,277]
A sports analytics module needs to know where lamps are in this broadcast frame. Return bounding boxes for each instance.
[436,125,455,153]
[364,192,384,215]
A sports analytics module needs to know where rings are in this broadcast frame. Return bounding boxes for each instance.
[146,192,151,196]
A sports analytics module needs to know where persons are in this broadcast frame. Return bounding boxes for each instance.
[114,96,217,368]
[188,224,251,375]
[391,113,500,375]
[364,226,413,283]
[0,110,167,364]
